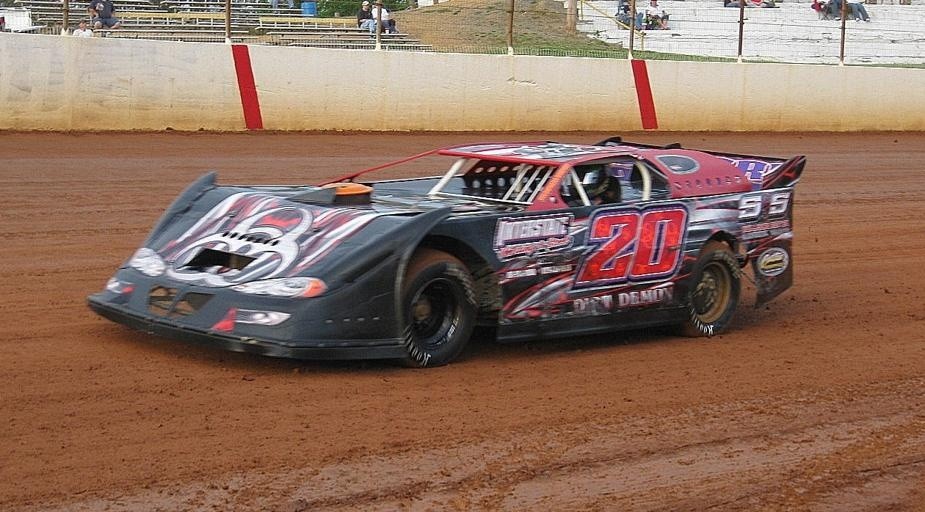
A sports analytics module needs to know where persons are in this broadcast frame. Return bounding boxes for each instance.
[72,19,95,37]
[588,175,622,206]
[371,0,395,34]
[724,0,778,8]
[88,0,123,37]
[617,0,671,31]
[811,0,870,22]
[270,0,297,17]
[356,1,377,39]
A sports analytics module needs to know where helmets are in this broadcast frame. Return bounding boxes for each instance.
[567,164,610,200]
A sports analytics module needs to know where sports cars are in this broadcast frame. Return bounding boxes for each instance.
[85,135,807,369]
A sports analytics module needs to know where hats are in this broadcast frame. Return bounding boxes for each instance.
[362,1,370,6]
[375,0,384,6]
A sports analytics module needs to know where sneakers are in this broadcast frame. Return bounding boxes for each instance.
[835,17,870,21]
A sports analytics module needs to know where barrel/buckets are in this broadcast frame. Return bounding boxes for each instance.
[301,0,318,18]
